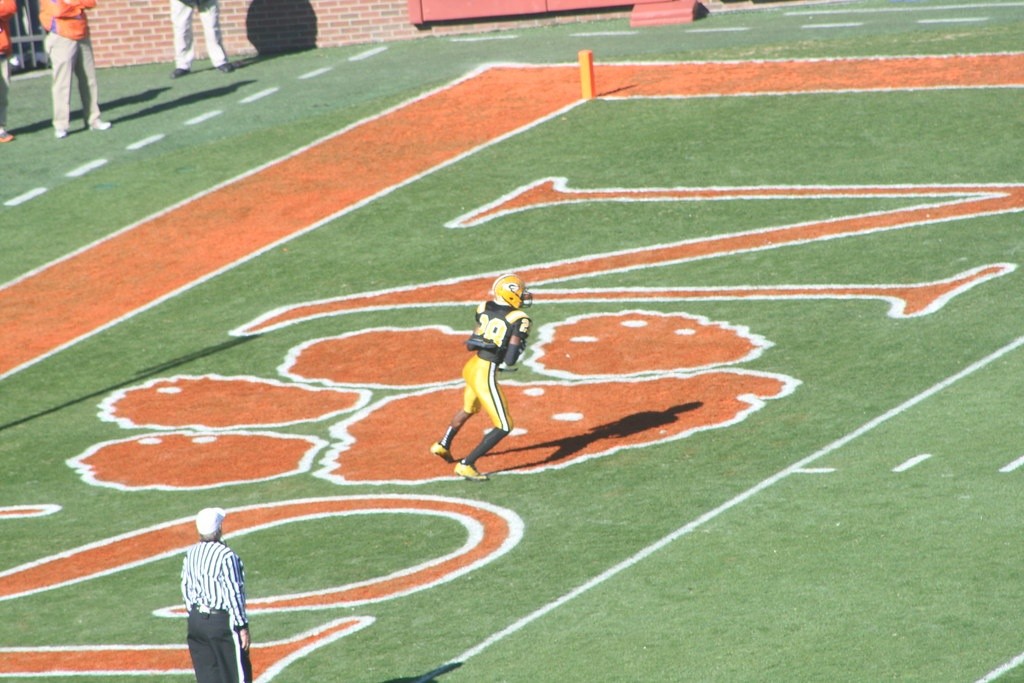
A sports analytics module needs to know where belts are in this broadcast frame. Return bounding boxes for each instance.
[194,605,226,614]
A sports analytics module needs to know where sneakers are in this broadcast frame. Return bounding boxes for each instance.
[455,461,489,480]
[430,442,455,463]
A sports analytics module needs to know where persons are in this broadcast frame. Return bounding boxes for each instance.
[170,0,235,80]
[180,507,252,683]
[430,274,534,480]
[39,0,111,139]
[0,0,18,144]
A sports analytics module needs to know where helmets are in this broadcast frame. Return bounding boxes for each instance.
[493,274,532,309]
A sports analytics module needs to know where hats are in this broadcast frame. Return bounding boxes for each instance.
[195,507,226,534]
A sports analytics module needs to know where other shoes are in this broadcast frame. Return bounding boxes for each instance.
[171,69,190,79]
[216,62,234,73]
[86,119,111,130]
[55,130,67,138]
[0,130,14,143]
